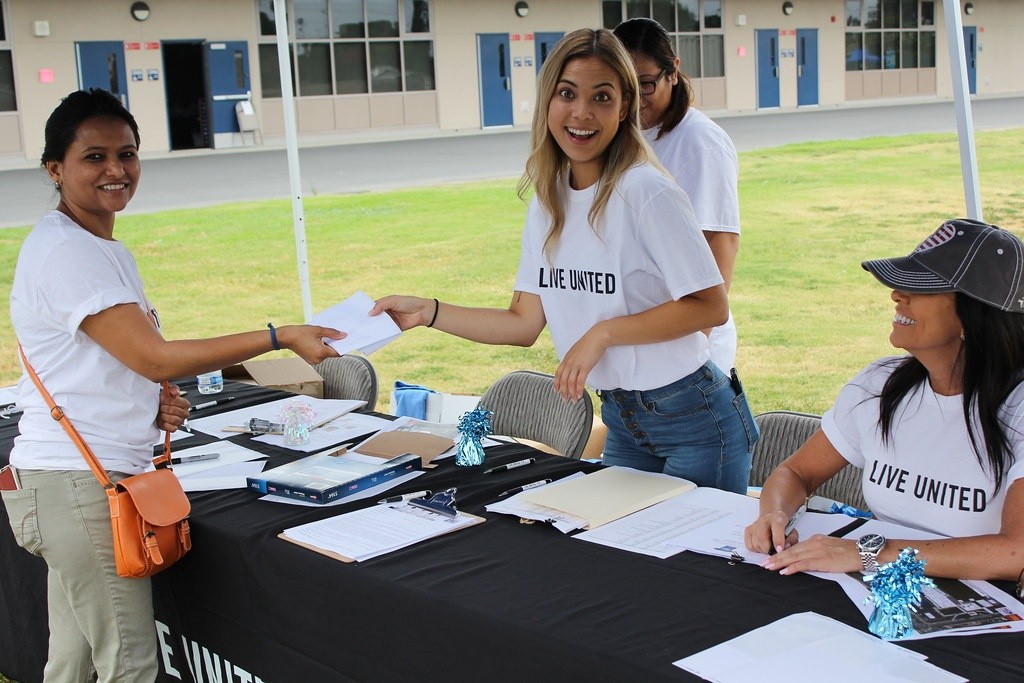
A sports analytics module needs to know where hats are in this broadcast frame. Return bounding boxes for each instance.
[861,219,1024,313]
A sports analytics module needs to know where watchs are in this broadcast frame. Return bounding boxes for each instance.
[856,534,887,571]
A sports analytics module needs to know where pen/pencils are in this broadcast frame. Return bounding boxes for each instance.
[500,478,552,497]
[172,453,219,465]
[377,490,434,504]
[483,458,537,474]
[188,396,236,412]
[768,504,809,556]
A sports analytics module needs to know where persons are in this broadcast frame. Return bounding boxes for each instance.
[611,17,740,377]
[745,219,1024,581]
[369,28,760,494]
[0,87,347,683]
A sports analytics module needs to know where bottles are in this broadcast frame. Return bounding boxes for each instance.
[195,369,223,395]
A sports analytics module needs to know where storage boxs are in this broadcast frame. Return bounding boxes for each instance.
[222,354,324,398]
[386,389,481,424]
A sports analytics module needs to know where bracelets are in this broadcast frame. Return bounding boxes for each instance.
[426,299,439,327]
[267,323,280,350]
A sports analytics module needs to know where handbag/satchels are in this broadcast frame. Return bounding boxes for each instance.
[105,469,192,578]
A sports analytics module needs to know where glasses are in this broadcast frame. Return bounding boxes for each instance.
[637,68,667,95]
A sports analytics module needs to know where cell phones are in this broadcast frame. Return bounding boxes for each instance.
[0,464,22,491]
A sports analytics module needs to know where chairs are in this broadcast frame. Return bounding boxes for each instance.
[471,370,594,459]
[747,410,873,518]
[311,354,379,413]
[0,376,1024,683]
